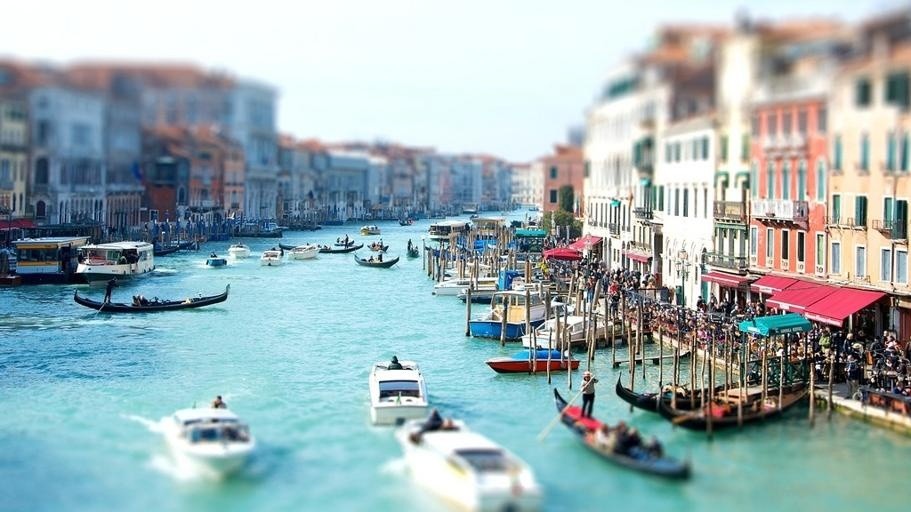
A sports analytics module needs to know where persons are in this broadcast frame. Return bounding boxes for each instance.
[425,409,453,429]
[388,356,403,370]
[104,277,148,307]
[593,421,662,461]
[211,395,227,410]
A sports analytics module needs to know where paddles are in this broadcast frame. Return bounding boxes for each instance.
[536,375,596,439]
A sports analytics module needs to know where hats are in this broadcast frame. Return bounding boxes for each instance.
[583,371,592,376]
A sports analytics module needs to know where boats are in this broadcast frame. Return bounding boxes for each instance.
[169,407,255,482]
[73,283,230,312]
[368,355,428,427]
[554,388,691,481]
[395,417,545,512]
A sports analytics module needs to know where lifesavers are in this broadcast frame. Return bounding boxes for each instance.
[543,237,549,247]
